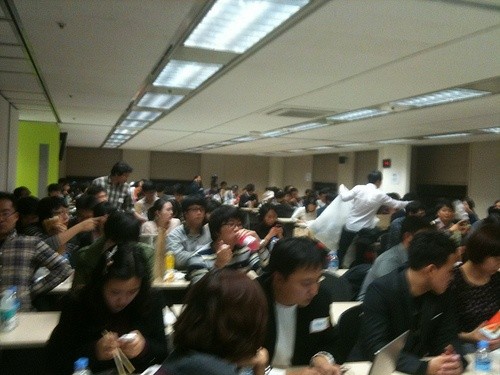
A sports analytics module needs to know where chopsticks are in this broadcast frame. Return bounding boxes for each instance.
[103,331,135,375]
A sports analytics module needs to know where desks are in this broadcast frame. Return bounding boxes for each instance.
[231,203,394,285]
[338,349,500,375]
[329,301,364,327]
[0,311,62,351]
[35,268,190,294]
[161,303,191,357]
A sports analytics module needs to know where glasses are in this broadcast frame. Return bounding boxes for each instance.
[51,208,69,216]
[185,207,206,213]
[0,211,19,220]
[222,223,243,231]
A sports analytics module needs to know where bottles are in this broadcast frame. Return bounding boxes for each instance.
[328,251,339,273]
[71,358,90,375]
[0,286,20,331]
[235,236,260,253]
[474,341,491,374]
[164,251,174,282]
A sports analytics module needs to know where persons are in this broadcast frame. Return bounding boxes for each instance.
[43,242,169,375]
[449,223,500,355]
[345,227,470,375]
[154,267,269,375]
[252,235,353,375]
[0,158,500,314]
